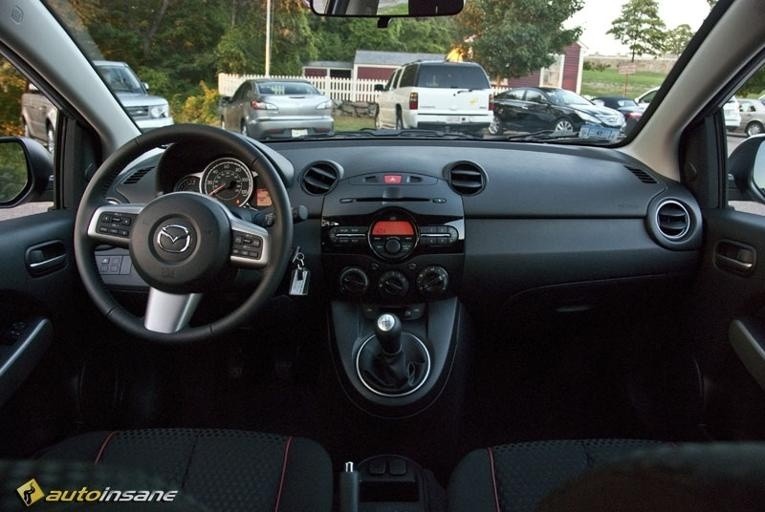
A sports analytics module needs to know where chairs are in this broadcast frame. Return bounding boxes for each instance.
[14,430,333,511]
[449,437,762,511]
